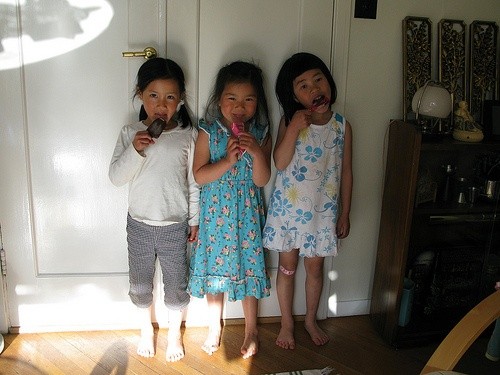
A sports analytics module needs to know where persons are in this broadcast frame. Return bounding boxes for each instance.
[458,101,473,130]
[108,57,201,363]
[262,53,353,349]
[485,318,500,361]
[184,62,272,360]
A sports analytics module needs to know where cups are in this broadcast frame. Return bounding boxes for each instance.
[465,181,480,204]
[484,178,500,200]
[453,177,469,204]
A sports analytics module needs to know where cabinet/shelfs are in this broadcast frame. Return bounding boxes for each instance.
[368,116,500,349]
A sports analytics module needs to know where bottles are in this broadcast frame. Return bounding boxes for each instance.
[437,163,455,205]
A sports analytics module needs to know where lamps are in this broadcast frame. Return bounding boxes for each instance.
[412,80,452,132]
